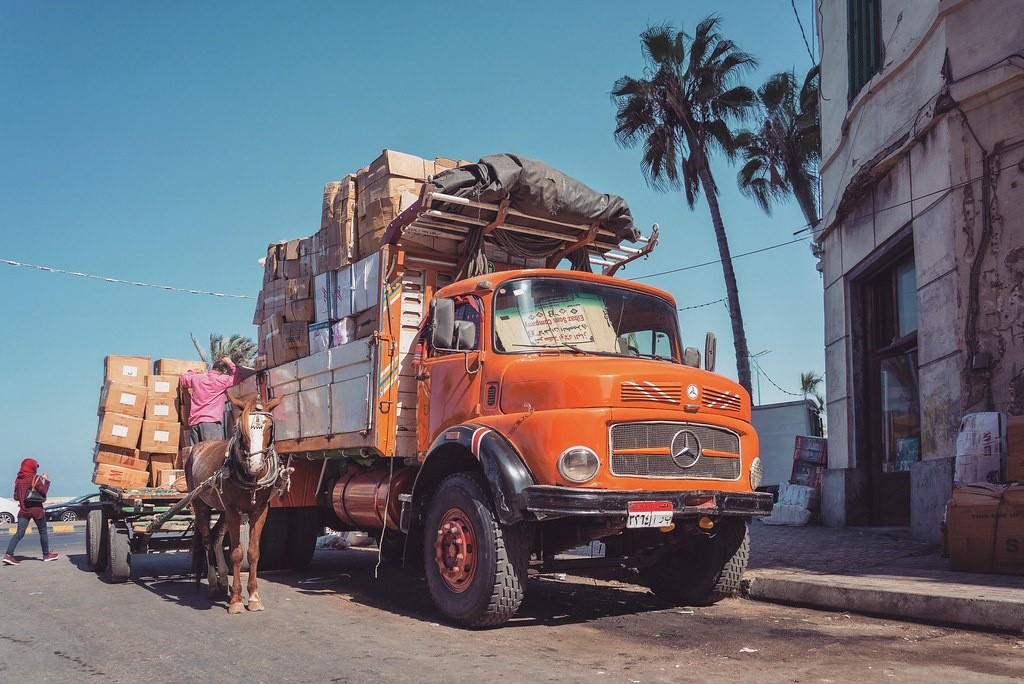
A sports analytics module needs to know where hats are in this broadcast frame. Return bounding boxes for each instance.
[222,357,236,373]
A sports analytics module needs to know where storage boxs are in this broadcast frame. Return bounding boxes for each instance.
[790,436,828,488]
[90,356,244,490]
[944,415,1024,574]
[252,150,547,372]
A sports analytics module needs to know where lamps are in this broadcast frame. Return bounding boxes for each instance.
[813,410,820,414]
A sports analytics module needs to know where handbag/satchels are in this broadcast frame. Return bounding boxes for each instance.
[24,475,47,502]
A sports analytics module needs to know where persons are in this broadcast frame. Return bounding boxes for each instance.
[179,357,243,449]
[2,459,60,565]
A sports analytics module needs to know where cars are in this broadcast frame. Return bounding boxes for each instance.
[43,493,100,521]
[0,497,19,524]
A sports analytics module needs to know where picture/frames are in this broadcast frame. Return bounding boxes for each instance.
[895,438,921,473]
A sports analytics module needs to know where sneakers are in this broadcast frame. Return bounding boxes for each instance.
[3,555,20,565]
[43,552,59,562]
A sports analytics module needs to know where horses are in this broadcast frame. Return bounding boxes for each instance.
[185,394,284,614]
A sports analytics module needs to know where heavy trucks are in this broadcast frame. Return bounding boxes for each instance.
[224,187,773,631]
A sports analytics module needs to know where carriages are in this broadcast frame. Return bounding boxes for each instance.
[86,389,294,614]
